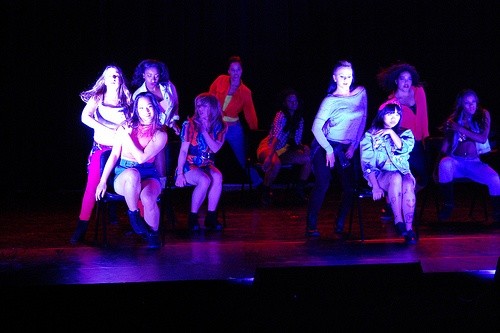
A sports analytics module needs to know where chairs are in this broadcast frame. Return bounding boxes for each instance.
[348,148,419,241]
[96,150,164,246]
[432,148,489,222]
[173,147,227,227]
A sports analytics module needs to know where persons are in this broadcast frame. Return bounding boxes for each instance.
[359,99,420,245]
[170,93,227,233]
[95,92,169,250]
[303,61,368,238]
[70,65,140,243]
[256,93,314,208]
[132,60,182,225]
[378,64,430,224]
[436,90,500,224]
[207,56,264,192]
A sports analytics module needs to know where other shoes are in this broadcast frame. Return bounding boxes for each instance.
[69,209,224,250]
[306,215,418,244]
